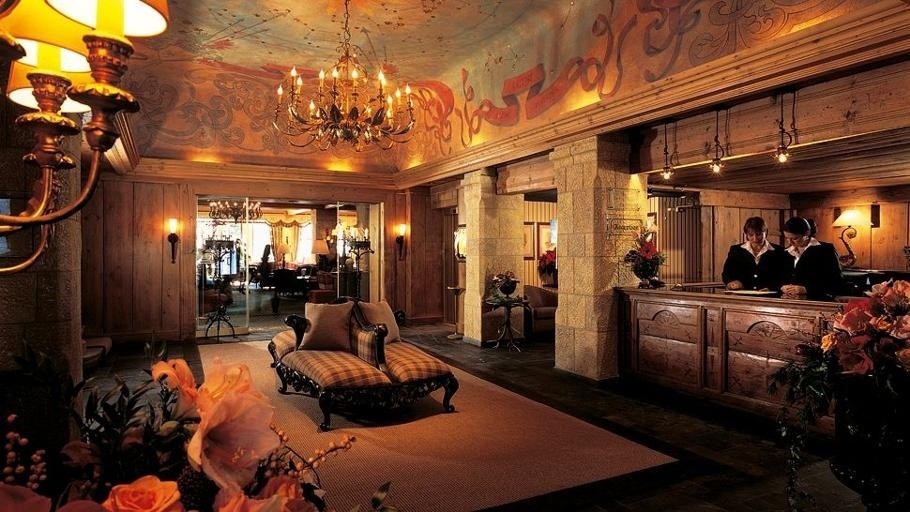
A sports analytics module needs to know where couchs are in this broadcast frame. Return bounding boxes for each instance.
[268,296,460,431]
[524,284,558,333]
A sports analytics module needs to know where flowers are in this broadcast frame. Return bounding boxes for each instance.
[539,247,556,274]
[493,271,515,290]
[769,278,910,480]
[1,359,397,512]
[626,225,667,280]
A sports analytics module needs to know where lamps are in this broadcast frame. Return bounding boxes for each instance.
[209,201,262,224]
[278,244,290,268]
[777,94,792,163]
[167,218,179,263]
[661,122,674,179]
[392,224,407,260]
[709,111,724,173]
[312,239,330,265]
[273,0,416,151]
[832,210,874,266]
[0,0,169,272]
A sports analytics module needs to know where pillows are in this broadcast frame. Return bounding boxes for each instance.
[358,300,402,344]
[300,302,354,353]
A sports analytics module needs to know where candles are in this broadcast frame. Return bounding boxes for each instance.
[364,228,369,239]
[353,228,357,239]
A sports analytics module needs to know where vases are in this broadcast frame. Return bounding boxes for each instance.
[635,278,656,288]
[550,272,557,285]
[499,283,517,297]
[830,462,910,512]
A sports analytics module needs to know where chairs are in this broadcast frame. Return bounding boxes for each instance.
[247,265,261,290]
[271,269,314,316]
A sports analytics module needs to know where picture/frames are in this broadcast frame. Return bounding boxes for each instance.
[524,222,535,260]
[537,222,551,260]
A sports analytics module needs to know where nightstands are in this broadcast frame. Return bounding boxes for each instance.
[308,290,337,304]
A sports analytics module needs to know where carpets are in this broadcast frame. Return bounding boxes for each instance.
[199,339,678,511]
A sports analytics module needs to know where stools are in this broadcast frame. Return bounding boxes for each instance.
[446,286,465,339]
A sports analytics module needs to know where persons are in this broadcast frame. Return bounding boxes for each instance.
[723,216,785,292]
[780,216,845,297]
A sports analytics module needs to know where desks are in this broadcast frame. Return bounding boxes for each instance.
[486,297,527,352]
[262,274,314,290]
